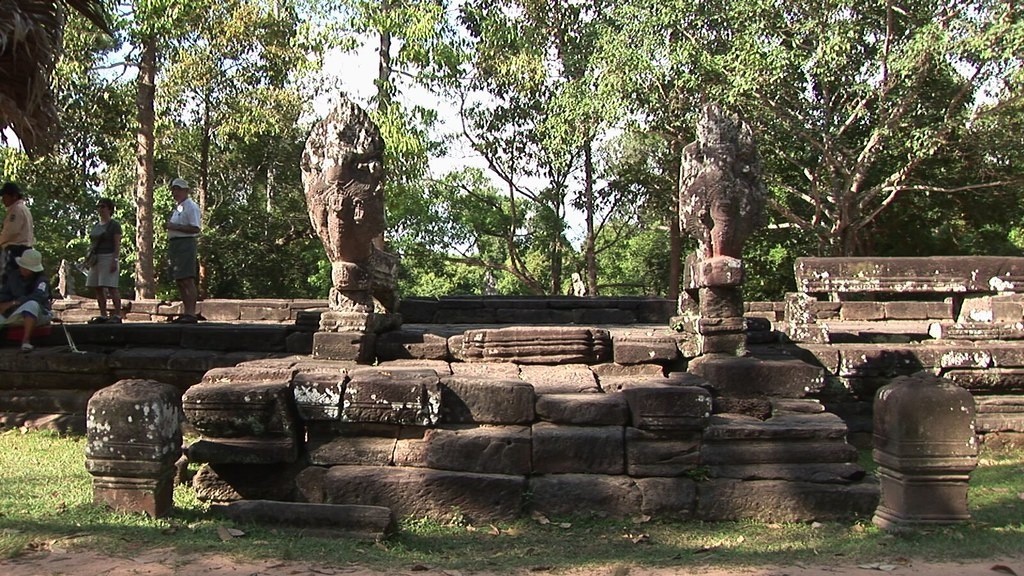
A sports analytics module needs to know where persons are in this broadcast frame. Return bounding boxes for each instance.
[85,198,123,324]
[162,178,201,322]
[0,182,51,353]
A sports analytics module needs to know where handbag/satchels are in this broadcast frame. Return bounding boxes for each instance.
[84,251,97,267]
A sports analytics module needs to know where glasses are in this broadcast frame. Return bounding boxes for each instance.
[95,203,109,209]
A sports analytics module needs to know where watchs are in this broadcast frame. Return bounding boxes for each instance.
[114,257,118,262]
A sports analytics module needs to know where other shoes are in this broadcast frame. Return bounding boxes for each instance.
[179,315,198,324]
[106,315,122,324]
[21,343,33,351]
[174,314,184,323]
[88,316,108,324]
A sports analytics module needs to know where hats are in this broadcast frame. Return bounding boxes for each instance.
[14,249,44,272]
[169,178,191,191]
